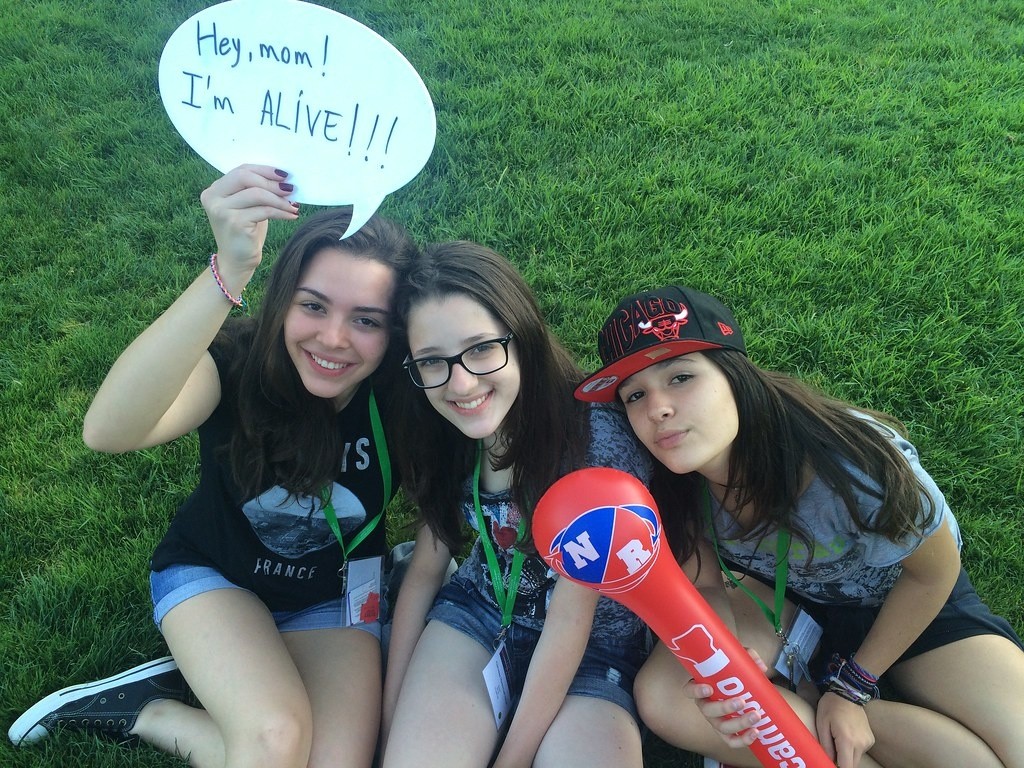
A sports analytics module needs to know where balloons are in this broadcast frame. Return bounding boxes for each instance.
[532,468,837,768]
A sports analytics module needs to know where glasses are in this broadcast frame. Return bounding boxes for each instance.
[402,331,515,389]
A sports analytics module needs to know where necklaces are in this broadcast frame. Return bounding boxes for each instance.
[715,474,745,511]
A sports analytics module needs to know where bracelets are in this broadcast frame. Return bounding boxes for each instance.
[825,650,881,707]
[210,253,243,305]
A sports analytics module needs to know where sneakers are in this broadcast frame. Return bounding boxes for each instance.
[8,655,190,747]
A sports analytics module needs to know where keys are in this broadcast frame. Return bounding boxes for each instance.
[786,654,794,691]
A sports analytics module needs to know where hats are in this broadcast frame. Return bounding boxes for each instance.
[573,285,747,403]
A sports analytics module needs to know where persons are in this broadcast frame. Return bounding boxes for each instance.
[379,244,687,768]
[8,164,419,768]
[574,285,1023,768]
